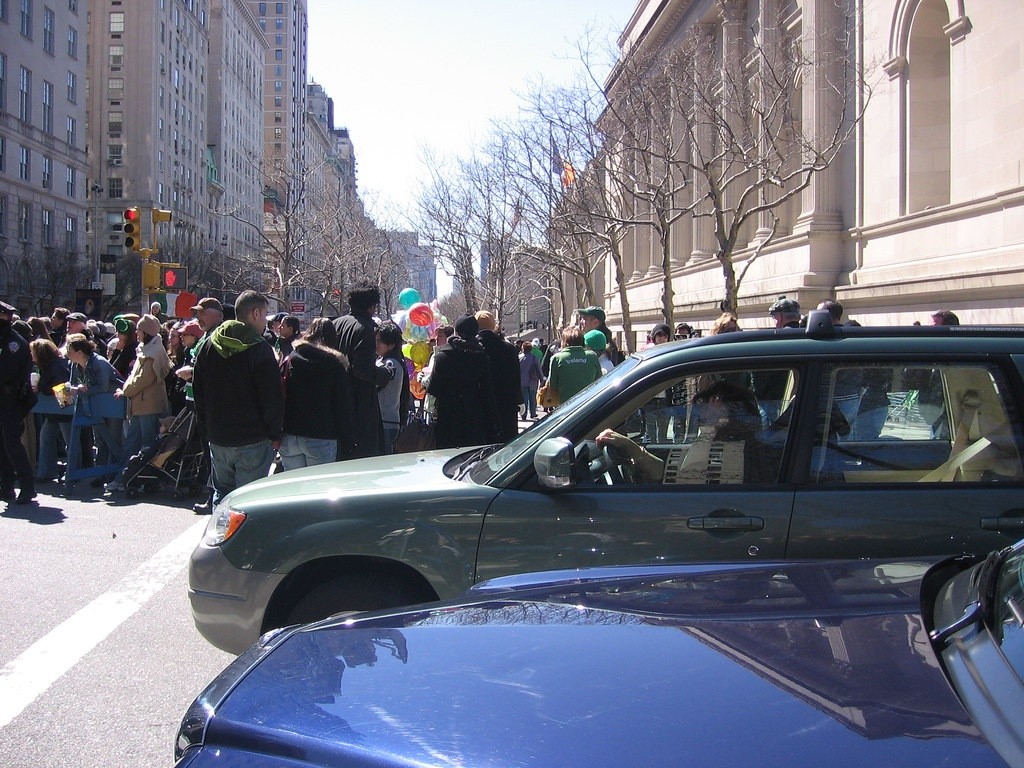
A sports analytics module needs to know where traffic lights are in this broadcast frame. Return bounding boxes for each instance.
[161,264,187,289]
[152,208,172,223]
[124,207,140,252]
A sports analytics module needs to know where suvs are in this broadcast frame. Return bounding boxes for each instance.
[187,306,1024,652]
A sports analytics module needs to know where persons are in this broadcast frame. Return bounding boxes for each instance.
[0,297,302,516]
[192,289,284,515]
[278,318,350,472]
[331,279,396,461]
[376,298,961,455]
[596,381,762,485]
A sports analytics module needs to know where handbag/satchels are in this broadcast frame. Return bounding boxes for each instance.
[393,389,437,455]
[536,379,560,407]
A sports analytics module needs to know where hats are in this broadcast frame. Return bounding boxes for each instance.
[652,324,670,343]
[531,338,539,346]
[768,295,801,317]
[475,311,496,329]
[177,321,203,338]
[693,373,741,404]
[578,306,606,322]
[584,329,606,350]
[65,311,88,324]
[158,415,176,426]
[189,297,223,312]
[10,320,34,339]
[455,313,478,339]
[151,302,161,310]
[137,314,160,337]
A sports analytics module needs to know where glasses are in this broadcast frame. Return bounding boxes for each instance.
[695,396,708,407]
[675,334,690,339]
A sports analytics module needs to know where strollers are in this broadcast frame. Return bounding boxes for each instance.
[121,384,195,503]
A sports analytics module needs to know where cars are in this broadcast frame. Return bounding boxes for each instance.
[168,536,1024,768]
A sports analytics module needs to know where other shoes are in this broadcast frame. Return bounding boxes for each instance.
[104,481,124,491]
[1,486,37,504]
[192,501,209,514]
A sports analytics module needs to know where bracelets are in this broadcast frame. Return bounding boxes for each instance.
[631,445,647,465]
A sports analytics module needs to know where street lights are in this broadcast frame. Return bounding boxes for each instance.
[91,183,104,281]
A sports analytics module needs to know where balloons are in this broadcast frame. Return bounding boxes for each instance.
[399,287,421,310]
[408,302,434,327]
[409,340,432,366]
